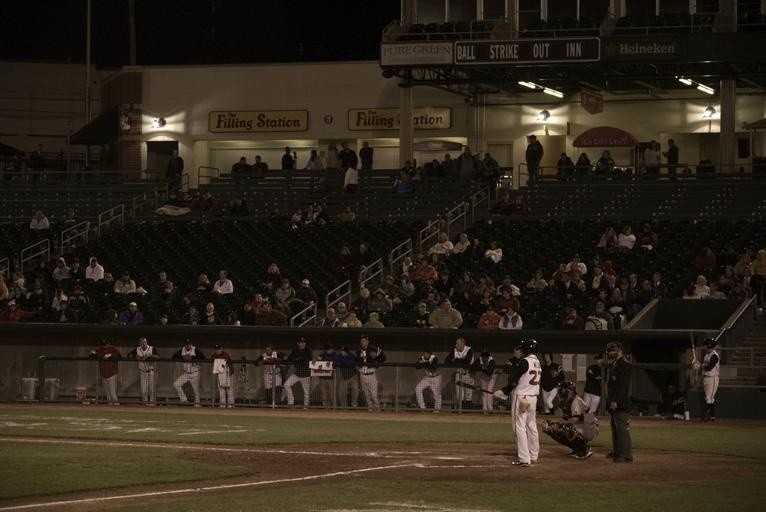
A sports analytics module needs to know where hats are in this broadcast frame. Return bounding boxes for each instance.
[480,349,490,357]
[130,301,137,307]
[518,336,540,355]
[605,341,624,365]
[702,337,718,349]
[419,302,427,309]
[593,352,603,360]
[303,279,311,286]
[327,307,334,313]
[420,345,432,357]
[98,334,370,354]
[337,302,346,309]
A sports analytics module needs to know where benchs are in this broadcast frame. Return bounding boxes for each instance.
[0,172,766,329]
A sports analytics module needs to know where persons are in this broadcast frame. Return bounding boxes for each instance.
[492,335,634,464]
[209,344,236,409]
[89,337,122,405]
[444,337,475,413]
[254,335,387,414]
[470,351,499,414]
[690,338,721,423]
[127,335,160,405]
[415,348,443,413]
[171,337,206,409]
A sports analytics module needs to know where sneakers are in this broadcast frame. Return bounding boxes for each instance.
[569,448,634,463]
[511,454,539,467]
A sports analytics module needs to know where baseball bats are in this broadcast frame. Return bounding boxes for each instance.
[456,380,493,394]
[690,333,696,358]
